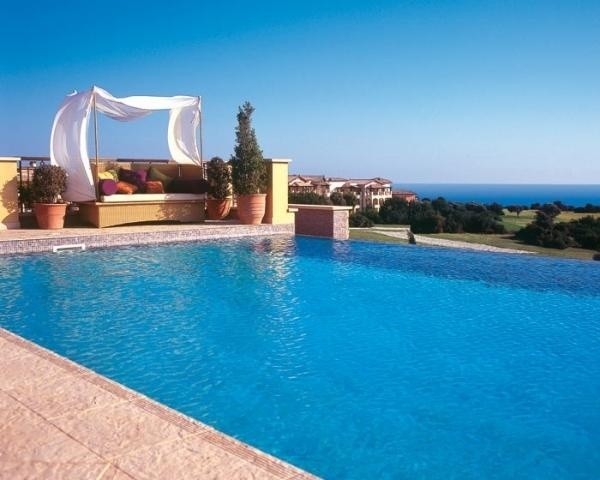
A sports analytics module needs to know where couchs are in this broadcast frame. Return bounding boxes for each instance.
[77,162,205,229]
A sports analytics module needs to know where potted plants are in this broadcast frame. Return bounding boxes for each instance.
[205,156,231,220]
[29,164,67,229]
[228,100,269,224]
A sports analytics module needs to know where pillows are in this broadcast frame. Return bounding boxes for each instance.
[98,165,208,196]
